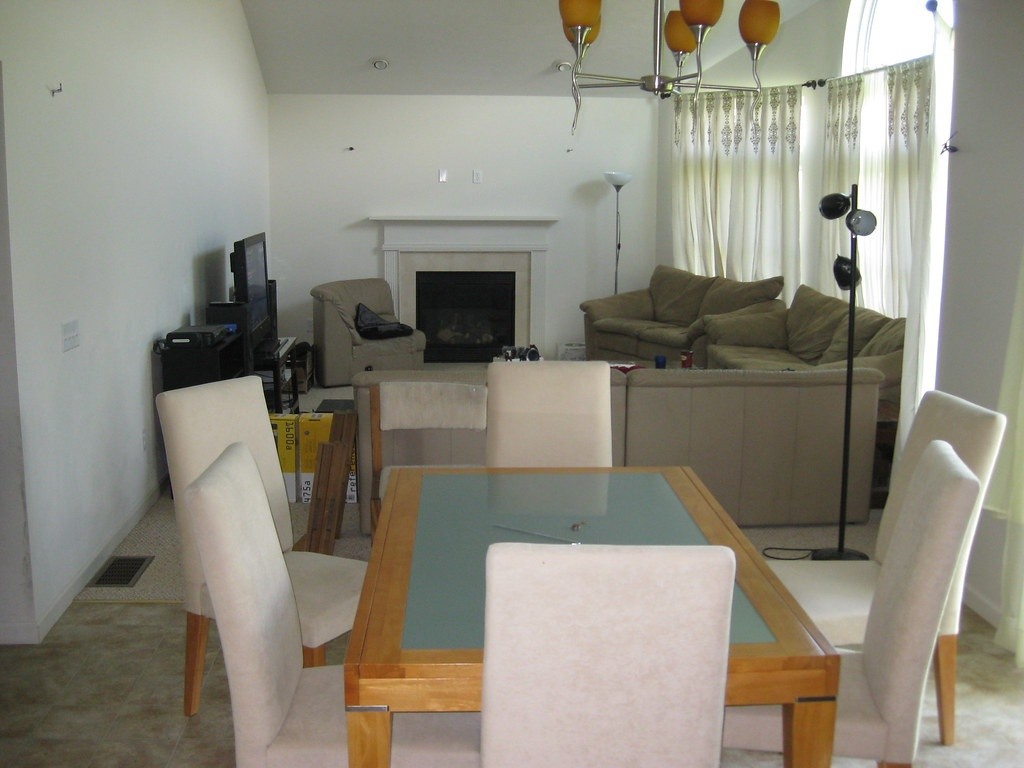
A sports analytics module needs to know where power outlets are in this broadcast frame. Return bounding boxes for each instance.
[473,169,481,183]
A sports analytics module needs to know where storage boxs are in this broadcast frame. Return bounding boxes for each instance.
[298,413,358,504]
[267,414,297,505]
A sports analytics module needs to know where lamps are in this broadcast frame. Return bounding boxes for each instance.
[555,0,780,136]
[602,171,633,295]
[814,184,878,560]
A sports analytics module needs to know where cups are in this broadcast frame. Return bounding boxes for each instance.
[654,355,667,369]
[681,350,694,369]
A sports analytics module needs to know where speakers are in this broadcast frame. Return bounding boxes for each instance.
[206,302,255,380]
[255,279,280,354]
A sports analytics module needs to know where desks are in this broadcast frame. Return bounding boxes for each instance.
[347,465,842,768]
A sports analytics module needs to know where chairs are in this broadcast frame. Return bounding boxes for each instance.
[188,442,480,768]
[723,441,982,768]
[487,361,611,468]
[159,376,370,718]
[483,543,736,768]
[822,390,1008,745]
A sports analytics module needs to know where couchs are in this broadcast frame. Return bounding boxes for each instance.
[310,278,426,387]
[579,263,786,370]
[353,369,625,535]
[626,367,885,526]
[705,283,907,403]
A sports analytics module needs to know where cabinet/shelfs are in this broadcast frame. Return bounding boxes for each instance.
[160,333,247,499]
[258,337,300,414]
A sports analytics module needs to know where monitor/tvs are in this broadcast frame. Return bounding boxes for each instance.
[230,232,273,356]
